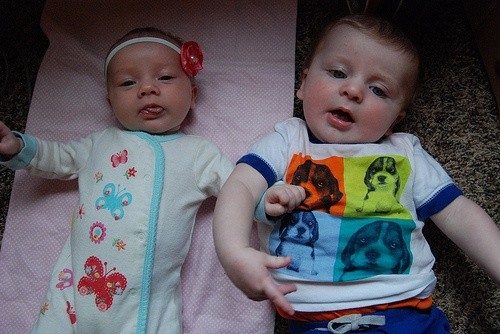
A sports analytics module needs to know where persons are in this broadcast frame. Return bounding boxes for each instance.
[213,13,500,334]
[0,28,306,334]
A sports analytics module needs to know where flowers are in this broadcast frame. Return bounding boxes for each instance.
[179,40,203,78]
